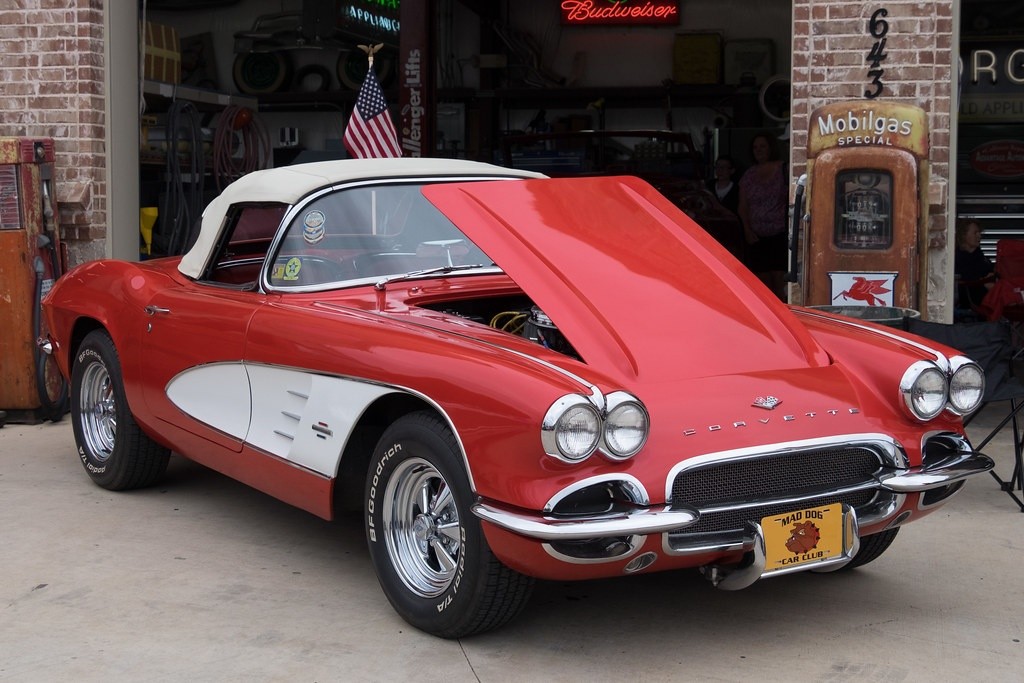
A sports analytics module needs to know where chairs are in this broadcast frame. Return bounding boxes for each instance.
[902,316,1024,514]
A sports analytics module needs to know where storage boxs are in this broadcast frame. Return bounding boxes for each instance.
[671,33,724,84]
[723,38,776,87]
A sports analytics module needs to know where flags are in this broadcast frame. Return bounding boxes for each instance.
[341,69,403,160]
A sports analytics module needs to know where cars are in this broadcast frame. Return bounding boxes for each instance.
[40,156,989,643]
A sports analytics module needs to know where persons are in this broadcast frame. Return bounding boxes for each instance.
[954,219,999,323]
[695,128,789,300]
